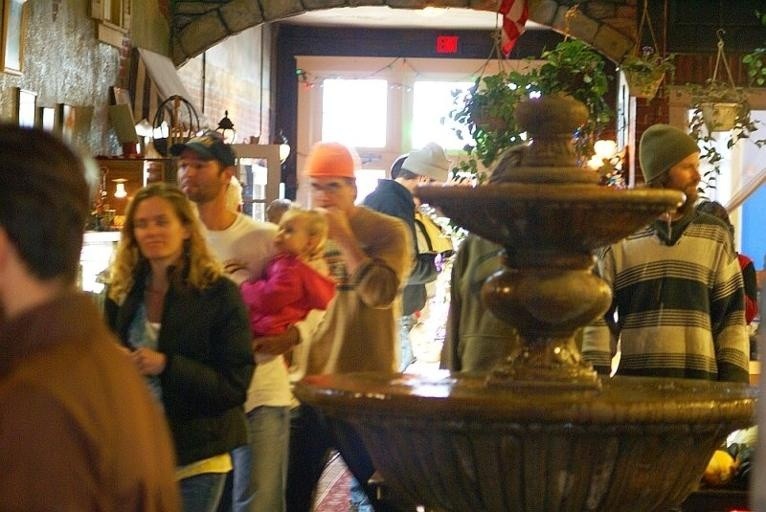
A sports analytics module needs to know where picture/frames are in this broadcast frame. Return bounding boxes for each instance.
[2,0,28,76]
[41,106,56,133]
[111,84,136,126]
[17,86,38,129]
[59,102,77,144]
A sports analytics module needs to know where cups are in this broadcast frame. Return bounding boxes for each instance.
[102,208,116,226]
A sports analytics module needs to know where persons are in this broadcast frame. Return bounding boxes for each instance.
[578,122,751,385]
[102,182,259,512]
[224,204,329,340]
[1,121,183,512]
[168,128,329,511]
[435,141,530,375]
[286,139,416,511]
[358,140,453,375]
[263,198,293,225]
[695,200,758,325]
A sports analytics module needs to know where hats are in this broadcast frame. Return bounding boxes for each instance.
[400,141,452,183]
[305,142,355,180]
[167,128,235,167]
[638,122,701,183]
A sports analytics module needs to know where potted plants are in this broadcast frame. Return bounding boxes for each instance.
[619,45,678,101]
[444,69,550,242]
[683,78,766,206]
[520,37,618,163]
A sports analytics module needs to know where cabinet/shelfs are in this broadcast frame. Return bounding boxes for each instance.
[76,228,122,295]
